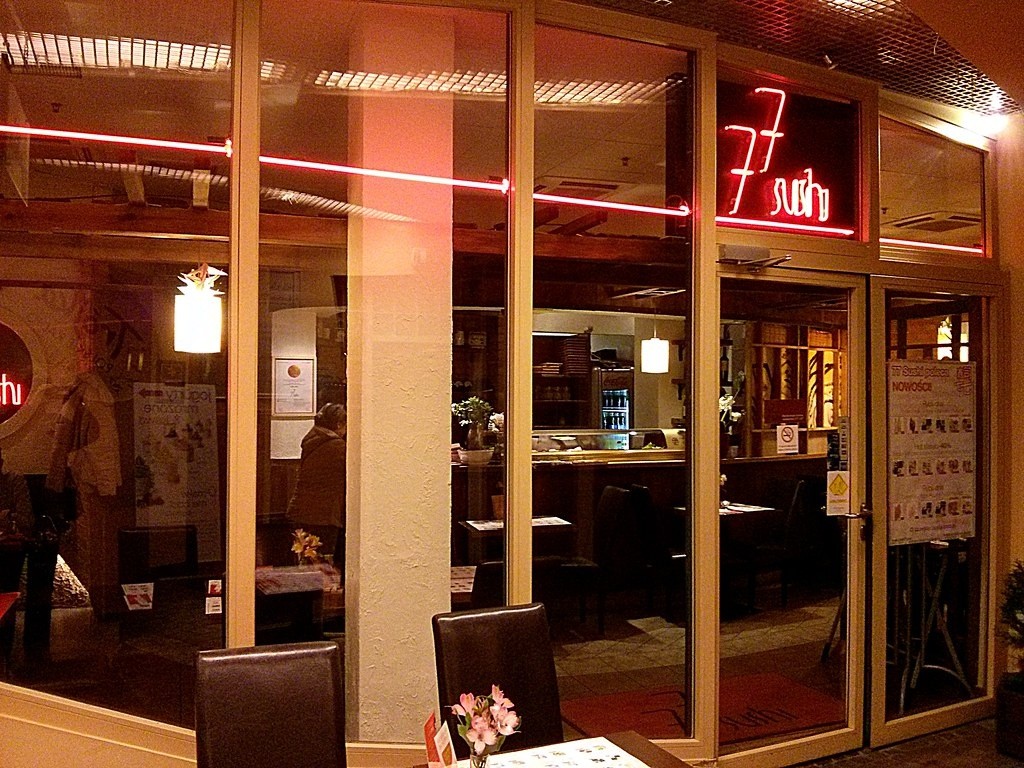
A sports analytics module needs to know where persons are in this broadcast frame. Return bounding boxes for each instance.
[0,383,99,682]
[284,403,348,641]
[0,452,34,541]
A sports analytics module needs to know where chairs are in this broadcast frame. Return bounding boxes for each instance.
[118,476,826,768]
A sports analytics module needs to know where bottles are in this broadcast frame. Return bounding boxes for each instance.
[602,392,626,430]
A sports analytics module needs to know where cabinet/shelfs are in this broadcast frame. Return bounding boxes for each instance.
[530,330,633,433]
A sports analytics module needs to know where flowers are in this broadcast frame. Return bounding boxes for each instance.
[292,529,323,564]
[444,684,522,768]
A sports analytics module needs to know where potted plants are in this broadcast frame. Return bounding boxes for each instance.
[452,396,495,466]
[995,563,1024,759]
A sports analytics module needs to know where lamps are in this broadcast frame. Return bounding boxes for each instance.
[641,314,669,374]
[173,241,228,353]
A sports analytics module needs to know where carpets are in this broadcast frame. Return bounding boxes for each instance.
[560,671,844,750]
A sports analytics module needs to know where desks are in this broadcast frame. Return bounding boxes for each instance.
[413,729,692,768]
[675,499,783,576]
[458,515,576,604]
